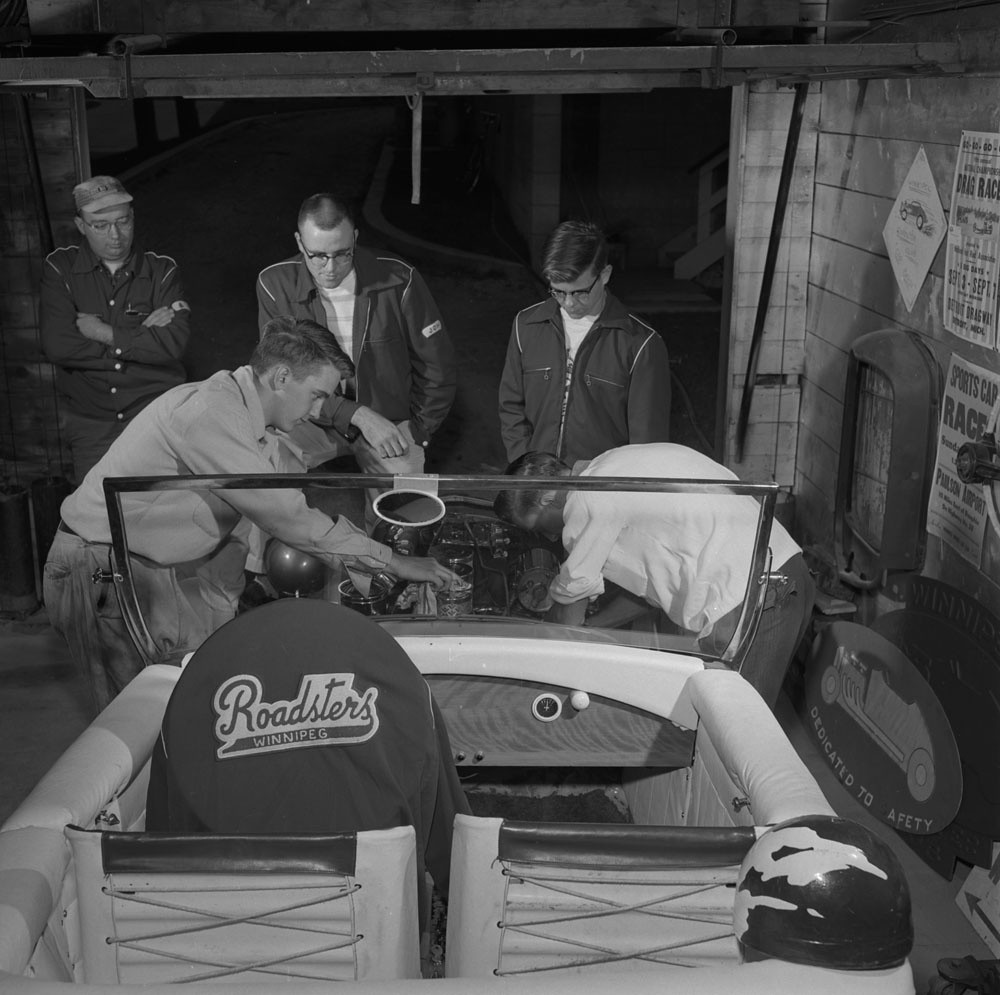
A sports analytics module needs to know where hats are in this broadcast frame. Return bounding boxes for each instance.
[74,175,134,212]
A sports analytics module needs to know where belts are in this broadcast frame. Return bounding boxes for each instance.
[58,520,80,536]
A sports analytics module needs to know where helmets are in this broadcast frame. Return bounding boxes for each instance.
[731,813,915,969]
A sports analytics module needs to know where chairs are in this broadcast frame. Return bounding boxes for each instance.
[144,597,467,881]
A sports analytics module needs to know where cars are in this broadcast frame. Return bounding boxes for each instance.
[0,474,918,995]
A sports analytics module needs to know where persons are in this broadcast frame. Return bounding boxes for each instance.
[255,192,458,475]
[38,174,192,503]
[497,218,673,631]
[42,314,467,716]
[492,441,819,714]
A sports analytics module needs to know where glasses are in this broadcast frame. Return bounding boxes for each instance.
[549,276,600,300]
[301,234,355,266]
[82,218,133,234]
